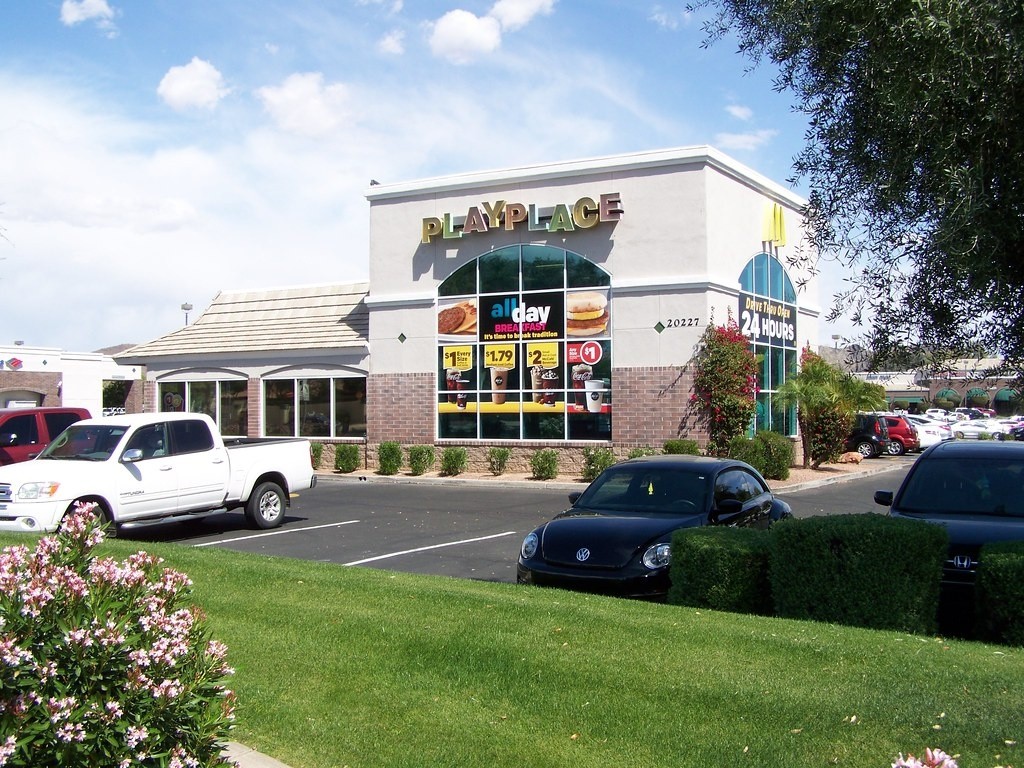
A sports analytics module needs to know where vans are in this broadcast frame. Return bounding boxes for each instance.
[0,408,92,465]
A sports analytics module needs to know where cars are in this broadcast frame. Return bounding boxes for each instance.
[999,421,1018,426]
[953,420,1005,440]
[911,419,941,447]
[907,416,951,439]
[1009,416,1023,420]
[516,455,792,600]
[1009,421,1024,440]
[875,439,1024,625]
[925,407,997,422]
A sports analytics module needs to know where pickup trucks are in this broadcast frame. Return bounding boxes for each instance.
[0,412,316,538]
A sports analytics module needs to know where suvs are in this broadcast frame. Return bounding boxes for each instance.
[879,416,919,454]
[839,416,887,459]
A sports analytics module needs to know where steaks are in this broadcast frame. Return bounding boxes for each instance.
[438,307,466,334]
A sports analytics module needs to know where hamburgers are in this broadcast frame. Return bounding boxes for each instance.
[565,293,609,336]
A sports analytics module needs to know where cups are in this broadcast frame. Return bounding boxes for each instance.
[446,369,461,403]
[542,378,558,407]
[571,365,593,411]
[531,372,543,402]
[456,380,470,409]
[584,380,603,412]
[490,367,507,404]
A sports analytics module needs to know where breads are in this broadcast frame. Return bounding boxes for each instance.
[450,300,477,333]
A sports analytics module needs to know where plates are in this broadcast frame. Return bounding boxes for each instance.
[438,303,477,341]
